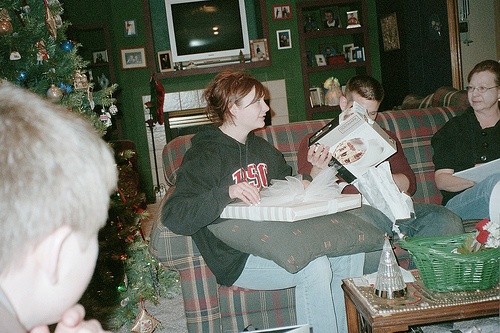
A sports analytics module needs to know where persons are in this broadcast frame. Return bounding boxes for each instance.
[0,82,118,333]
[323,10,339,28]
[295,75,464,269]
[430,60,500,227]
[127,54,142,64]
[96,53,104,64]
[280,34,289,47]
[159,71,366,333]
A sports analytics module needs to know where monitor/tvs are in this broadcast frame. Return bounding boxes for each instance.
[164,0,251,62]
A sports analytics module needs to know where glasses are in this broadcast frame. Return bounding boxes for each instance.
[465,84,500,94]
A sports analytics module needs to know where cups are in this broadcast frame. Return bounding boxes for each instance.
[336,55,345,65]
[328,56,337,65]
[175,62,182,71]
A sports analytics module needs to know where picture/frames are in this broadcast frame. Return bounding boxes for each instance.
[343,43,365,63]
[315,54,327,66]
[276,29,292,50]
[250,39,270,62]
[321,7,343,31]
[91,49,109,64]
[120,46,147,70]
[123,19,138,37]
[272,4,293,22]
[158,50,176,72]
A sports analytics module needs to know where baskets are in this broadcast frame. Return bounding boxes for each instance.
[401,233,500,293]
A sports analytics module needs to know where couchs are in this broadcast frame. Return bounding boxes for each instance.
[148,88,485,333]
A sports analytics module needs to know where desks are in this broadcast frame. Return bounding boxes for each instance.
[341,269,500,333]
